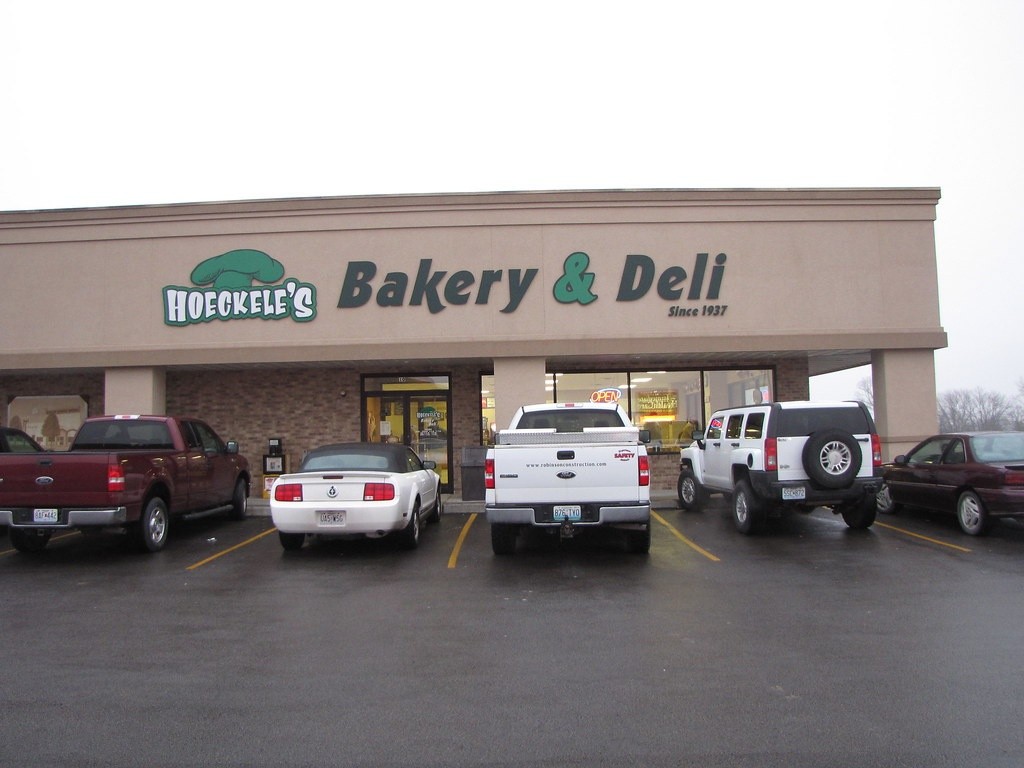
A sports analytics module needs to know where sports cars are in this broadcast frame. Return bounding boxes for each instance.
[267,443,442,552]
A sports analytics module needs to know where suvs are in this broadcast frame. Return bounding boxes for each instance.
[679,399,886,537]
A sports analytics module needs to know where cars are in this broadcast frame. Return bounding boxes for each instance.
[876,425,1024,536]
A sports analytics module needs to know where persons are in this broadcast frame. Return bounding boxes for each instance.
[679,416,697,443]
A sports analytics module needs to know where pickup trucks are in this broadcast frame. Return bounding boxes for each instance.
[483,397,652,559]
[1,412,250,554]
